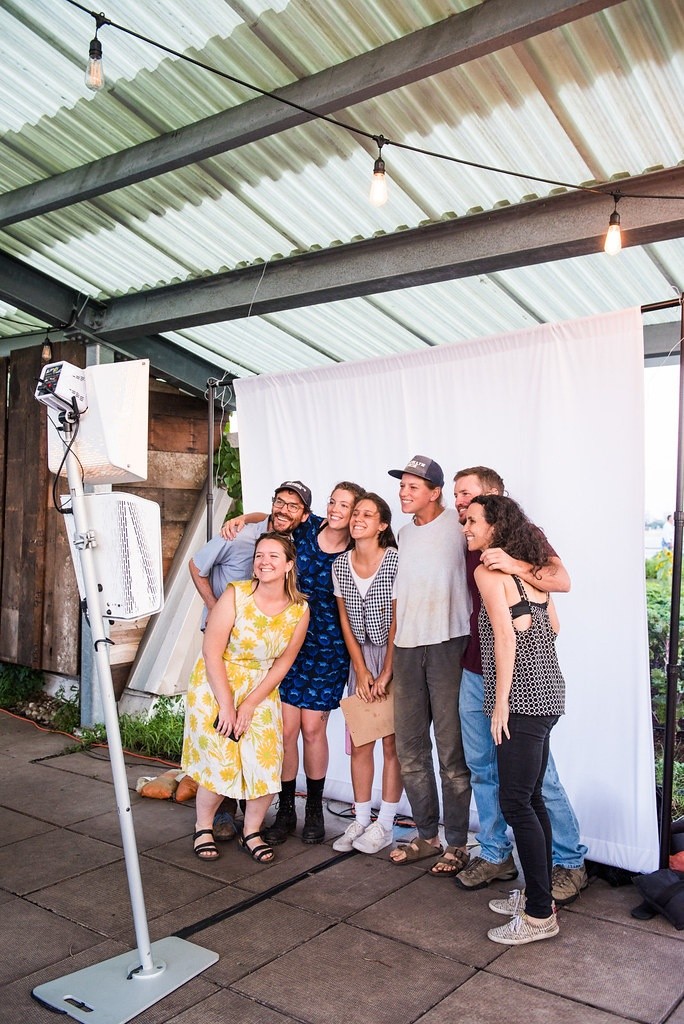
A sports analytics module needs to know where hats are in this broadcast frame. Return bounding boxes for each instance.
[275,480,313,510]
[387,456,445,487]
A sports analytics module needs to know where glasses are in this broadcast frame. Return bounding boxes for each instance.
[271,497,305,513]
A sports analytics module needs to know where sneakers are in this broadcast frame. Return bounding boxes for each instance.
[331,819,372,852]
[455,853,519,891]
[488,914,560,945]
[551,865,590,904]
[488,888,560,917]
[352,821,393,854]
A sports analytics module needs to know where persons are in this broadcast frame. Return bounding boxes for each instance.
[388,455,473,877]
[462,491,565,945]
[454,465,591,906]
[331,492,399,854]
[188,480,312,840]
[181,531,311,864]
[220,482,368,846]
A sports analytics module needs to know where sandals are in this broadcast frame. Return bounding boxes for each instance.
[237,831,275,863]
[389,837,444,867]
[428,846,471,877]
[192,829,221,861]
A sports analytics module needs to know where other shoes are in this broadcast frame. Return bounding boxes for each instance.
[213,812,237,840]
[301,803,326,843]
[264,800,297,844]
